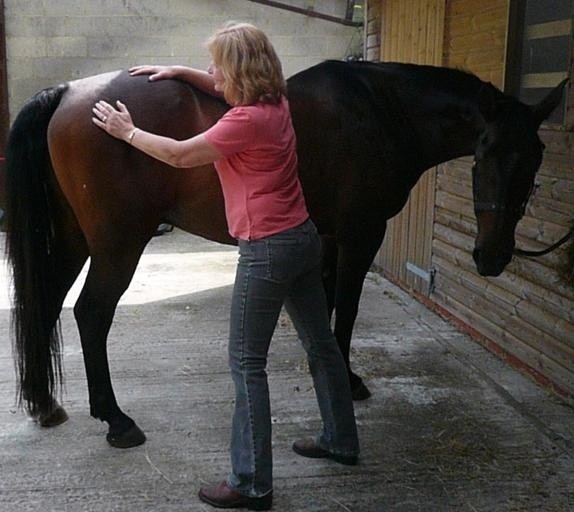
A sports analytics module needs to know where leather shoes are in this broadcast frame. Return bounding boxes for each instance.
[200,481,273,510]
[292,439,358,465]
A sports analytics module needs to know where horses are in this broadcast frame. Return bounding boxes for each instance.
[1,56,573,448]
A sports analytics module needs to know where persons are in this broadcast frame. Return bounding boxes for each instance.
[90,20,362,510]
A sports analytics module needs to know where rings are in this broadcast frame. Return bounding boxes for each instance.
[102,117,107,122]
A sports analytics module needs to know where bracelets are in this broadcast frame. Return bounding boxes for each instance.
[127,128,139,144]
